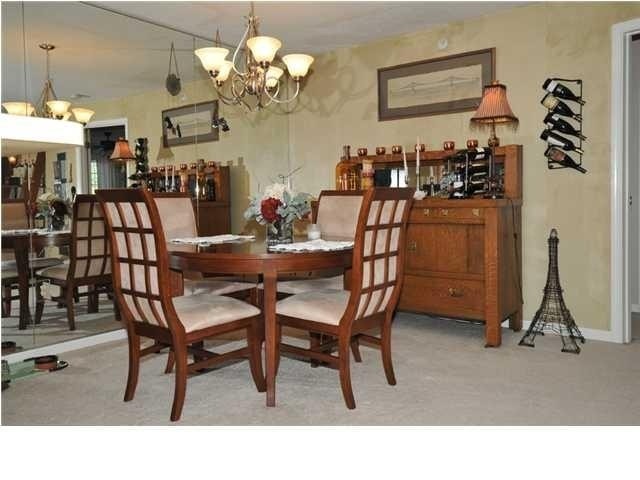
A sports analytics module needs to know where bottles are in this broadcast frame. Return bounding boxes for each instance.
[544,146,587,174]
[540,129,584,155]
[542,77,585,107]
[444,147,492,163]
[443,163,490,176]
[540,92,583,122]
[543,112,587,142]
[446,182,492,195]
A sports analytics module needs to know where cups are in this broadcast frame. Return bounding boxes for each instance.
[307,224,321,240]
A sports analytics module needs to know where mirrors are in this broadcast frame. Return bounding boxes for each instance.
[374,163,446,188]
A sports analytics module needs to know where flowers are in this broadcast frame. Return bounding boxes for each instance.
[244,183,315,233]
[35,192,72,218]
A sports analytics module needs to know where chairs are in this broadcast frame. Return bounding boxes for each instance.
[33,193,122,330]
[257,189,366,367]
[274,186,415,410]
[149,191,256,375]
[93,186,266,421]
[2,198,63,323]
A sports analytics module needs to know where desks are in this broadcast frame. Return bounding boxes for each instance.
[1,228,71,330]
[165,234,355,408]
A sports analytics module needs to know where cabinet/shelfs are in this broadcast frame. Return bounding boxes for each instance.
[149,166,231,236]
[334,145,524,347]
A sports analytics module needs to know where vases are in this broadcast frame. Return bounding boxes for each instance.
[47,219,64,231]
[265,222,295,252]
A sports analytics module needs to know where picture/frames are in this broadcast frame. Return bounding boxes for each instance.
[161,99,219,148]
[377,47,496,122]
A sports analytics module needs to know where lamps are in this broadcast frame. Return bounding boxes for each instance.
[193,2,315,114]
[1,45,96,127]
[109,137,137,188]
[471,81,520,147]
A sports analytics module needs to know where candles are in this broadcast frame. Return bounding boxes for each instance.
[416,135,421,175]
[165,163,169,186]
[171,166,176,191]
[402,146,410,185]
[429,165,436,179]
